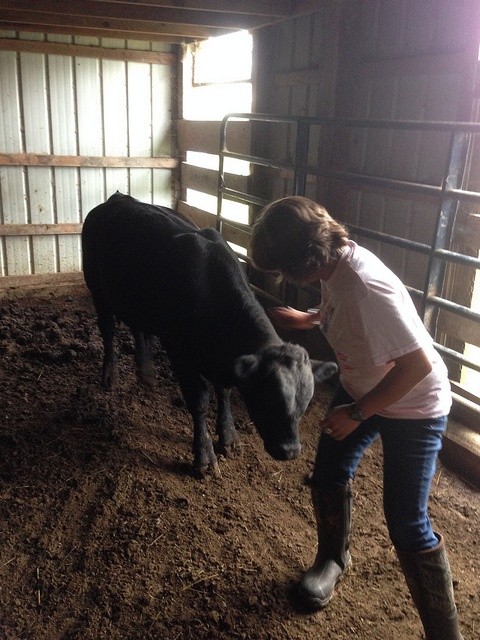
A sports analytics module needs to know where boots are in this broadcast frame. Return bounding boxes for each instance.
[299,479,353,608]
[397,531,462,640]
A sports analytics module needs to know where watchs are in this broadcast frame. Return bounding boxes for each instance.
[351,402,367,423]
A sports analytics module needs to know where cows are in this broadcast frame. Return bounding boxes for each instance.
[81,191,339,480]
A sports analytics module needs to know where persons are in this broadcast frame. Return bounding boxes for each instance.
[249,195,464,640]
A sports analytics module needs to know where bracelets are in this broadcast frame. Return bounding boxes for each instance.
[307,308,321,324]
